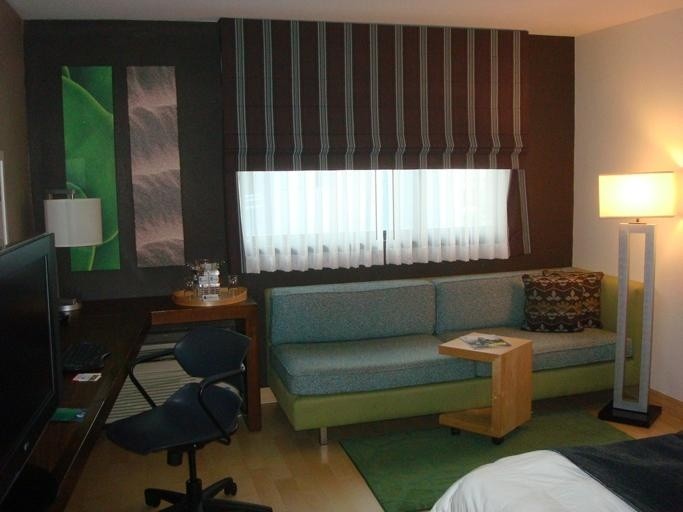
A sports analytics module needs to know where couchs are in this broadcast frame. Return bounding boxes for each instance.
[264,266,645,446]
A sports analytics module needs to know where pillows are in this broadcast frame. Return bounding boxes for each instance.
[522,273,584,332]
[542,269,604,329]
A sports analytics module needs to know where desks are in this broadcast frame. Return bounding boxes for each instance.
[0,312,151,512]
[60,265,262,431]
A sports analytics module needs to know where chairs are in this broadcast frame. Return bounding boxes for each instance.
[105,327,273,512]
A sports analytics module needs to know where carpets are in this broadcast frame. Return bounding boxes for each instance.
[338,403,635,512]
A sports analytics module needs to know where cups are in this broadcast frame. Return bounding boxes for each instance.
[183,256,240,302]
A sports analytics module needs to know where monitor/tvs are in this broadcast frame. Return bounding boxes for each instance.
[0,232,61,504]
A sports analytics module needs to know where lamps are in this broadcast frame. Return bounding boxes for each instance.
[598,171,676,428]
